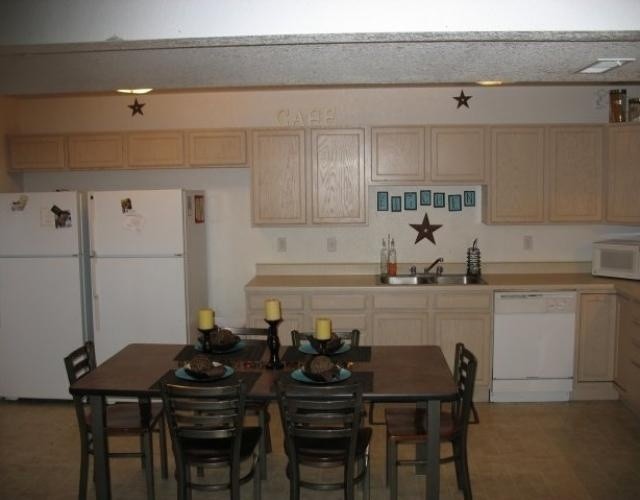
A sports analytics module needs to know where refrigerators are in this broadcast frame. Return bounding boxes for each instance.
[86,187,208,409]
[0,189,91,407]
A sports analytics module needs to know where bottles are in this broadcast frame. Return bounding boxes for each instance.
[380,238,388,277]
[389,237,397,276]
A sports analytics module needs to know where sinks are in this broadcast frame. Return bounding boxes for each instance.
[434,274,489,286]
[375,274,433,285]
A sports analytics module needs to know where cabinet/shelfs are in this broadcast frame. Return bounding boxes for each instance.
[307,289,374,346]
[126,127,185,170]
[483,123,546,226]
[435,291,492,389]
[244,285,307,348]
[575,288,616,387]
[547,123,605,227]
[186,127,250,168]
[249,126,369,228]
[606,122,640,224]
[65,129,126,170]
[370,126,490,184]
[616,287,639,416]
[374,290,435,346]
[7,129,66,170]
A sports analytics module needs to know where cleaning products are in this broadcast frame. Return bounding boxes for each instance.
[379,237,388,278]
[388,238,397,277]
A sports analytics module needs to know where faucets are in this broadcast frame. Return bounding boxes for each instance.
[424,257,444,274]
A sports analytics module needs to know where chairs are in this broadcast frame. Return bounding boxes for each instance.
[191,320,278,456]
[383,340,481,499]
[286,322,371,441]
[155,371,269,500]
[60,339,171,500]
[273,372,379,500]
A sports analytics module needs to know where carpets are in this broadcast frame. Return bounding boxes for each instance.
[366,389,484,430]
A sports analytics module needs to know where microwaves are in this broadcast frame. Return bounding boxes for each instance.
[591,237,640,281]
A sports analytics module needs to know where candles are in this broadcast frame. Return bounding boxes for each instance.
[264,298,280,320]
[316,318,332,341]
[199,308,215,330]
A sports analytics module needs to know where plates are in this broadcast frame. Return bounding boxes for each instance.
[298,341,353,356]
[174,363,235,382]
[193,340,246,354]
[291,369,352,384]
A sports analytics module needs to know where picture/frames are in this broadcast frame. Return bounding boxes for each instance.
[373,188,478,216]
